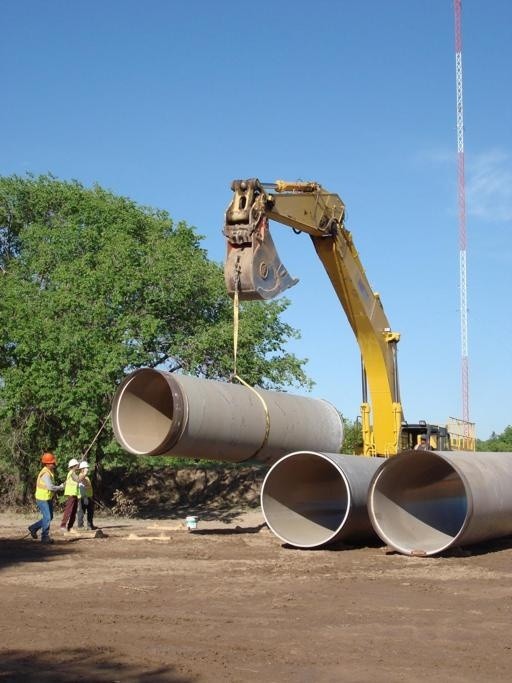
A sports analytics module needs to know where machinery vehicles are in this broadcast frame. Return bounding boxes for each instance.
[222,178,452,457]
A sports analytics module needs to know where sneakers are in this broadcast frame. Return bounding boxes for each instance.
[29,527,37,539]
[41,539,54,544]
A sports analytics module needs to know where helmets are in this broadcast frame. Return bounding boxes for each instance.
[42,453,55,464]
[68,459,79,468]
[79,461,90,469]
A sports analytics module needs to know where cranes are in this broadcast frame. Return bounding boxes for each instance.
[446,0,485,449]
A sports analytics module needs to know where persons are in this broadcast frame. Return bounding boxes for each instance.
[60,458,89,532]
[28,453,65,544]
[78,461,97,530]
[414,434,432,451]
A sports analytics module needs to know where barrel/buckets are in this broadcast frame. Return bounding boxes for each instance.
[185,515,199,530]
